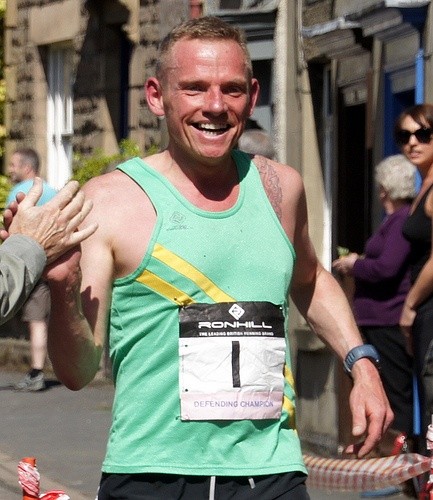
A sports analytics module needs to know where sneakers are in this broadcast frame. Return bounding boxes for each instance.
[14,372,46,391]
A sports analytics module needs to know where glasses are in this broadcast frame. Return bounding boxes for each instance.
[395,127,433,145]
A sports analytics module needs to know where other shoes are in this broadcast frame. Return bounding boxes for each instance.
[360,482,409,497]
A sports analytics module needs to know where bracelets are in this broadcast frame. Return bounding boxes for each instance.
[343,343,381,376]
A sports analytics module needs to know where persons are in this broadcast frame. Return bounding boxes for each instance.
[0,16,433,500]
